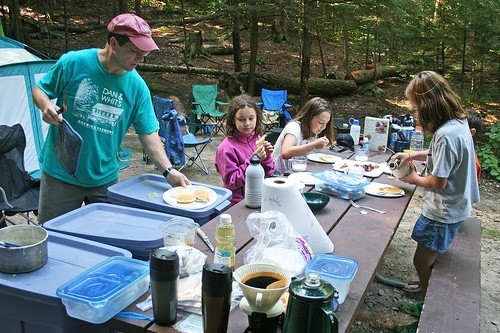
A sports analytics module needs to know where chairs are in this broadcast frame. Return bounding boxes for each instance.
[256,89,292,124]
[0,123,40,228]
[162,112,213,175]
[143,97,173,164]
[190,82,230,134]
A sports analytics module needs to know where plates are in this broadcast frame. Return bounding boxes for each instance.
[333,159,384,178]
[307,154,342,163]
[287,172,315,185]
[163,185,218,209]
[365,183,405,197]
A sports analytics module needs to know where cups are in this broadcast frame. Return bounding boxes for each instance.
[288,156,308,172]
[233,262,292,311]
[149,248,180,326]
[239,296,284,333]
[159,217,199,248]
[355,149,369,161]
[202,262,233,333]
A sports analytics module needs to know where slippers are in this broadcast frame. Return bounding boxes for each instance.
[402,281,422,292]
[394,321,419,333]
[399,297,424,318]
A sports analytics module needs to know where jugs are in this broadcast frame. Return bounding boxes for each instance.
[281,272,339,333]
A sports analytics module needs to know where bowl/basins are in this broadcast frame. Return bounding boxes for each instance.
[302,192,330,211]
[0,224,48,274]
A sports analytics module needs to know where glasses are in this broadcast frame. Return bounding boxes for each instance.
[116,38,149,57]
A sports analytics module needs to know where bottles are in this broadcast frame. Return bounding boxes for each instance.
[244,156,265,208]
[362,137,369,150]
[213,214,236,272]
[389,160,418,179]
[348,118,361,145]
[394,141,404,153]
[410,125,424,152]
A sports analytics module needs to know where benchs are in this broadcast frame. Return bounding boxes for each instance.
[416,216,483,333]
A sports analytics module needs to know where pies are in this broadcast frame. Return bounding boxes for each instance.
[255,135,267,147]
[382,186,401,193]
[175,189,210,204]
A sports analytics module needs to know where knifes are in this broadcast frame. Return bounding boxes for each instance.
[197,228,215,253]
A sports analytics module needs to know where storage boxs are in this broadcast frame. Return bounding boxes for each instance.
[306,255,358,306]
[310,168,369,201]
[42,200,195,263]
[0,227,132,333]
[56,255,151,324]
[106,172,233,228]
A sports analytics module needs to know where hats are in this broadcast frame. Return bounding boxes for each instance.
[107,13,160,52]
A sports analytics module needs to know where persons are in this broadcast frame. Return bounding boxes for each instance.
[273,97,333,175]
[391,71,480,333]
[33,14,193,223]
[214,94,276,206]
[403,110,483,292]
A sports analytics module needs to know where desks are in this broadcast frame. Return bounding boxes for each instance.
[76,136,428,333]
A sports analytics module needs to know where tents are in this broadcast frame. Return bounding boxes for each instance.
[0,35,59,179]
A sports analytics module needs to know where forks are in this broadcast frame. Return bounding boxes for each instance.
[349,199,387,214]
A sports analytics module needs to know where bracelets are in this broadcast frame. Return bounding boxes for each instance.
[163,167,174,177]
